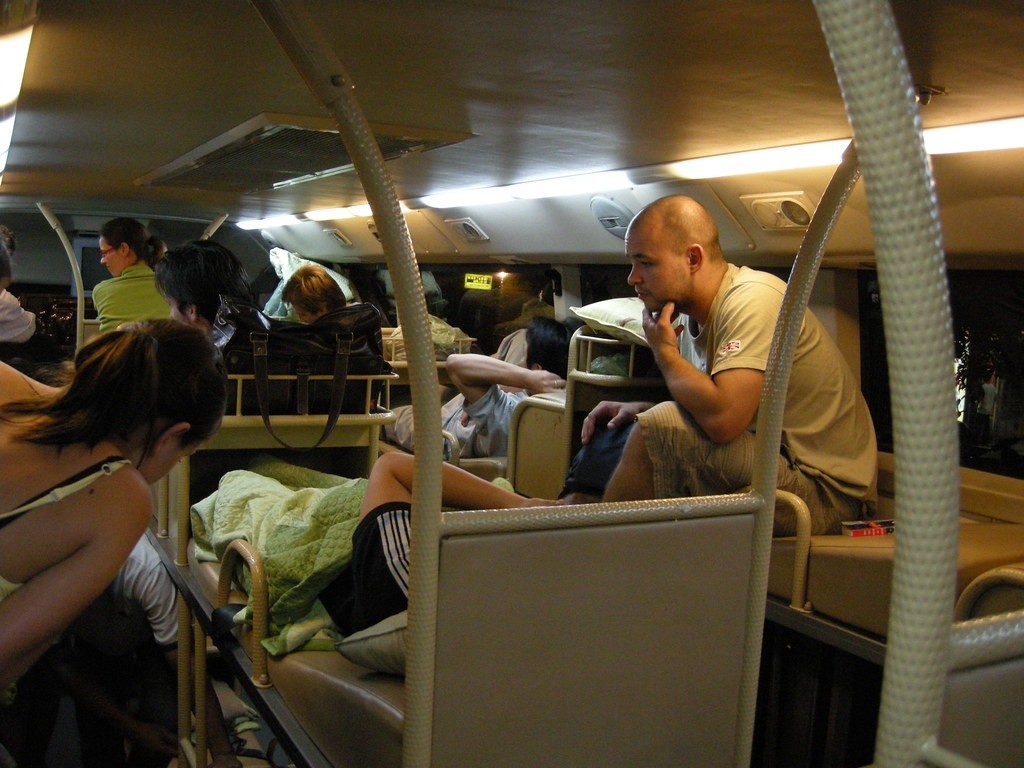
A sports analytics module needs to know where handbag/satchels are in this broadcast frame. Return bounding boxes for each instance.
[213,293,386,453]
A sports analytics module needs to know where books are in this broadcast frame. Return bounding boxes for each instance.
[840,519,895,537]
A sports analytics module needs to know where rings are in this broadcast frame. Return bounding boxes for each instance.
[554,380,558,388]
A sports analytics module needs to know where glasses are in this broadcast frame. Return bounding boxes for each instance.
[100,245,120,256]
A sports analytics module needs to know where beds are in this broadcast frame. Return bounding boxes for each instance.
[81,318,1024,768]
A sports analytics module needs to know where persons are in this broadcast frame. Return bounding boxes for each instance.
[0,217,586,768]
[970,372,996,449]
[557,194,879,538]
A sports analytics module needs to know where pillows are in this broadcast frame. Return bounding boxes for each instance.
[569,297,650,349]
[333,611,407,673]
